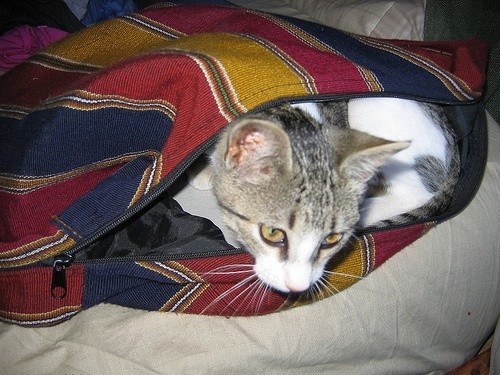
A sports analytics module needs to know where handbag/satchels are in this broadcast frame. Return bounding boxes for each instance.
[0,0,496,328]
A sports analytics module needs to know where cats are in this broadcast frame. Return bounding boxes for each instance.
[184,96,461,317]
